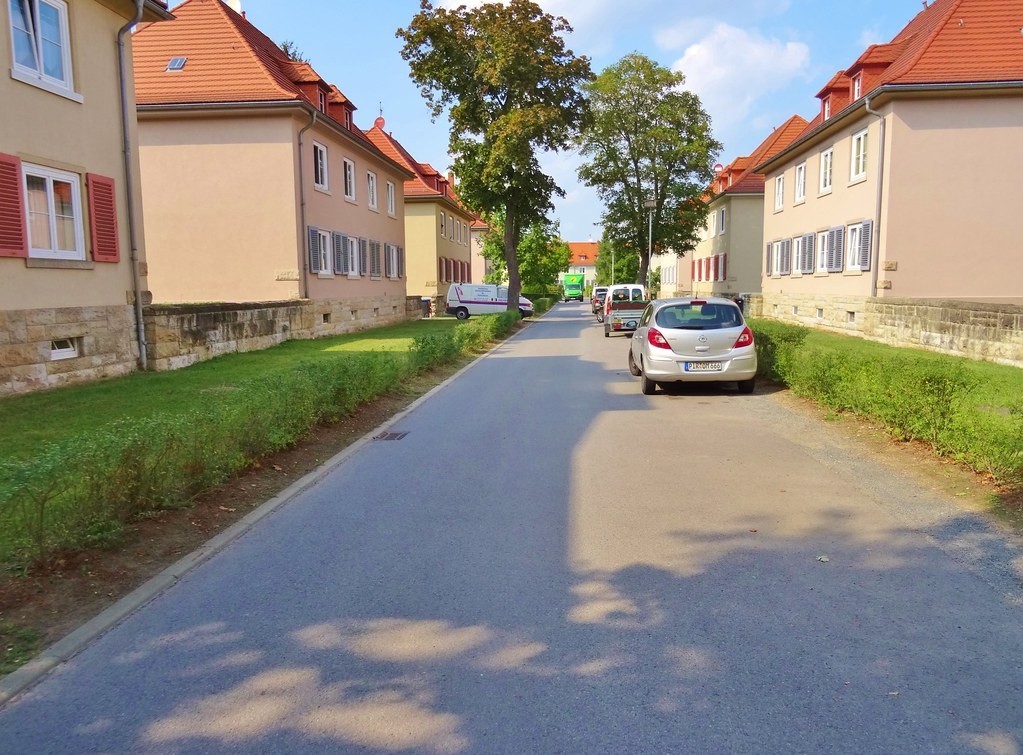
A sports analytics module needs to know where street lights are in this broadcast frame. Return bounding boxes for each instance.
[645,198,656,298]
[609,238,615,284]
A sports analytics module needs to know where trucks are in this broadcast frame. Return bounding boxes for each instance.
[564,274,585,302]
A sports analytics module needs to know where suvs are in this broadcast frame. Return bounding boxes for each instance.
[591,292,604,313]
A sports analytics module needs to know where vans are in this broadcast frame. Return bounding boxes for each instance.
[445,282,534,320]
[590,287,609,301]
[599,284,646,320]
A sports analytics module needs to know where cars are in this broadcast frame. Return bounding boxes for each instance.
[626,293,759,394]
[596,297,604,322]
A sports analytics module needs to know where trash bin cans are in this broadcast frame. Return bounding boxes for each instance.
[421,297,431,318]
[732,297,744,316]
